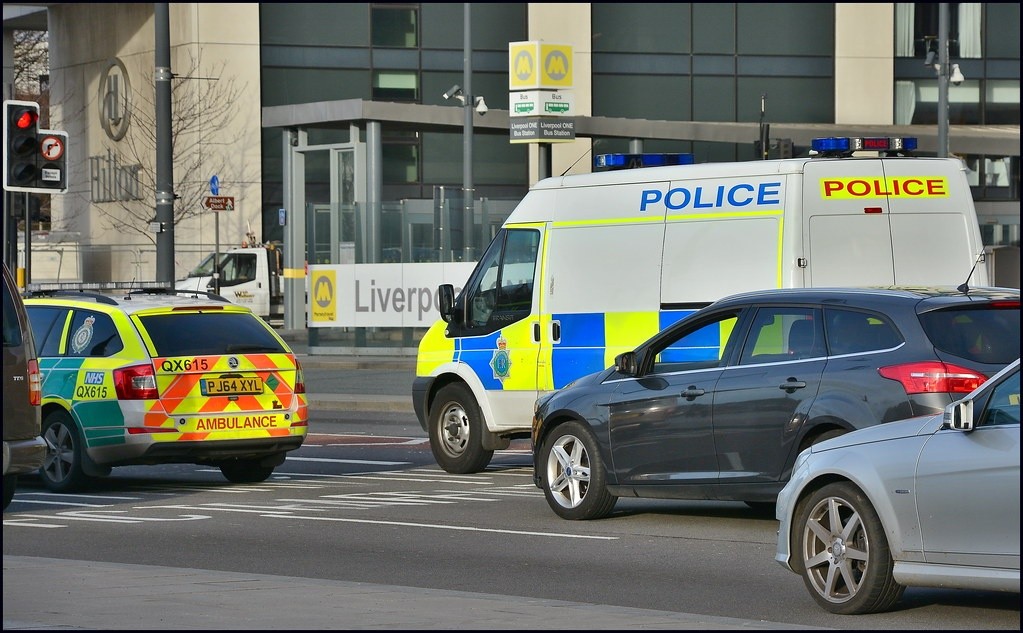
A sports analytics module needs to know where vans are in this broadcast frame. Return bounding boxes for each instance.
[408,131,992,475]
[1,261,47,513]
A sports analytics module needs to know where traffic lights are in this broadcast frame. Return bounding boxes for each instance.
[3,99,40,192]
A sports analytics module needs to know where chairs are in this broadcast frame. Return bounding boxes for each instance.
[790,319,813,353]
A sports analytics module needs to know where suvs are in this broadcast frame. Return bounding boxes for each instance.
[531,284,1018,520]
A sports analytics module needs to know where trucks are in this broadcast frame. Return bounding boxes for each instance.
[172,244,309,330]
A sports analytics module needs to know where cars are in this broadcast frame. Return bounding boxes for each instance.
[776,356,1023,617]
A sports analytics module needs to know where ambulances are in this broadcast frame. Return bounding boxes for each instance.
[18,286,309,495]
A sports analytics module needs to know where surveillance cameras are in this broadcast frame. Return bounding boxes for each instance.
[923,51,936,70]
[443,84,461,100]
[948,63,965,86]
[475,97,488,116]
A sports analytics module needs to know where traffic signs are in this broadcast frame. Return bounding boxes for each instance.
[200,195,235,212]
